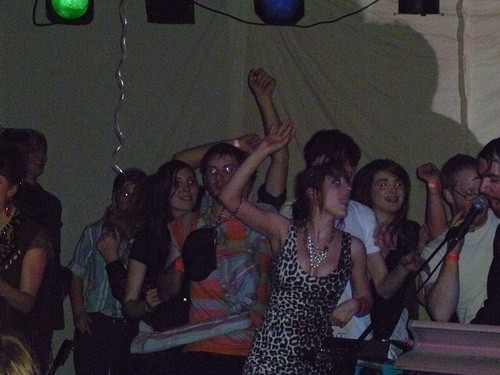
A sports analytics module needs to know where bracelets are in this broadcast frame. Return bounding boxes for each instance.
[445,252,459,260]
[356,297,371,318]
[427,181,440,188]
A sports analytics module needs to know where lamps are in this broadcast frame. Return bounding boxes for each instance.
[254,0,305,26]
[32,0,95,27]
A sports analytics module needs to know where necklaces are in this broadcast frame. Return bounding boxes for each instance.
[0,207,17,236]
[469,224,476,232]
[304,224,337,278]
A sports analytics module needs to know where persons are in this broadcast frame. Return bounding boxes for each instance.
[415,153,500,324]
[17,129,66,329]
[1,322,54,375]
[66,66,450,375]
[469,137,500,326]
[0,128,53,375]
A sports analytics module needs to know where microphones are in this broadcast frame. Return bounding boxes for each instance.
[449,195,488,252]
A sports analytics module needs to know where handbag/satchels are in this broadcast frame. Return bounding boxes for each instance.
[181,193,243,282]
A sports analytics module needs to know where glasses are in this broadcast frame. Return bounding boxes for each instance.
[205,166,236,175]
[117,189,132,198]
[453,185,483,201]
